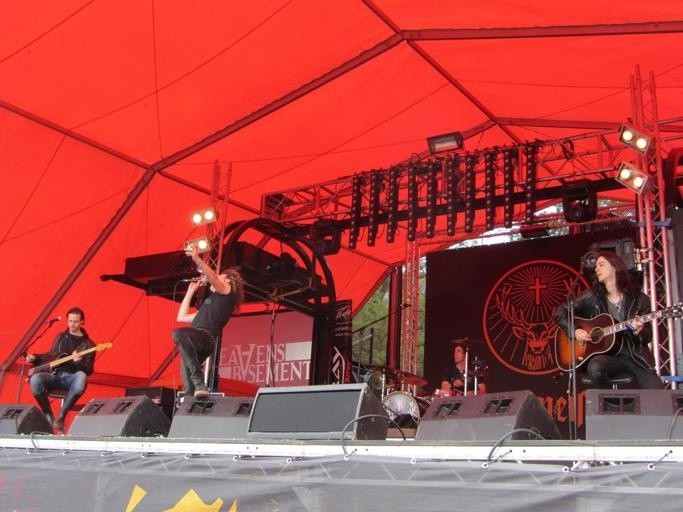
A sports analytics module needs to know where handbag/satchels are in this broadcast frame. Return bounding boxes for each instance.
[196,260,203,273]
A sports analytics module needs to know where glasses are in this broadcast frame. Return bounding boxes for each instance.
[613,160,653,197]
[183,236,210,257]
[189,206,218,228]
[617,121,656,158]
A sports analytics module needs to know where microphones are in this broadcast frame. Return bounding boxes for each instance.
[581,371,635,390]
[47,389,71,436]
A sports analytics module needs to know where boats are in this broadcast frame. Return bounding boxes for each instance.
[167,397,255,438]
[586,389,683,440]
[0,404,53,435]
[67,395,171,437]
[247,382,389,440]
[415,389,564,440]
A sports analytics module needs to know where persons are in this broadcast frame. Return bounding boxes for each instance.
[552,252,665,388]
[25,306,97,429]
[171,244,245,398]
[440,342,486,396]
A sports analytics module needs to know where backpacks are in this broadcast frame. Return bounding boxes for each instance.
[381,392,431,428]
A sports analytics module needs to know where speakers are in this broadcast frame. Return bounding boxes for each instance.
[33,390,54,426]
[52,390,80,430]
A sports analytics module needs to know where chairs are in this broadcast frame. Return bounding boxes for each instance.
[191,382,210,398]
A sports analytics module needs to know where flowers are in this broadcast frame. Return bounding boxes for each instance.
[182,278,205,282]
[581,259,596,265]
[45,317,61,324]
[403,303,412,307]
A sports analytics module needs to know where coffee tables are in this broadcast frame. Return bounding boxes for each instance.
[367,364,428,385]
[449,339,484,344]
